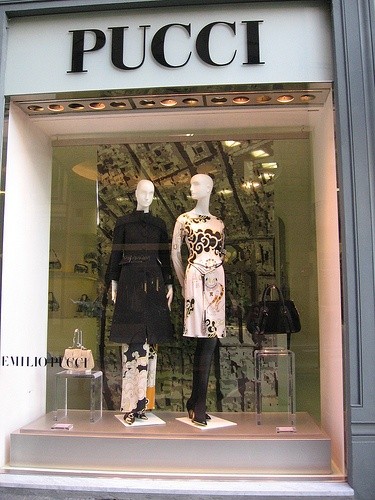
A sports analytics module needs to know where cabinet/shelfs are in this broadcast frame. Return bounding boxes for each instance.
[253,349,296,428]
[53,368,103,423]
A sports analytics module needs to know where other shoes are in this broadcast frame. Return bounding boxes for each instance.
[135,412,147,420]
[124,413,134,425]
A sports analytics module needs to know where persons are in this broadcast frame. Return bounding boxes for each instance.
[104,178,177,429]
[170,172,231,429]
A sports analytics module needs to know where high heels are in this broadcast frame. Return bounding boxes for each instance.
[187,398,211,420]
[192,402,208,426]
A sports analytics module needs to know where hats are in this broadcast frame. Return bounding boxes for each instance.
[84,253,99,270]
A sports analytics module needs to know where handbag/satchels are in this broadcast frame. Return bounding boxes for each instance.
[49,249,61,269]
[48,291,59,311]
[59,329,94,371]
[76,294,94,311]
[248,284,302,333]
[74,263,89,273]
